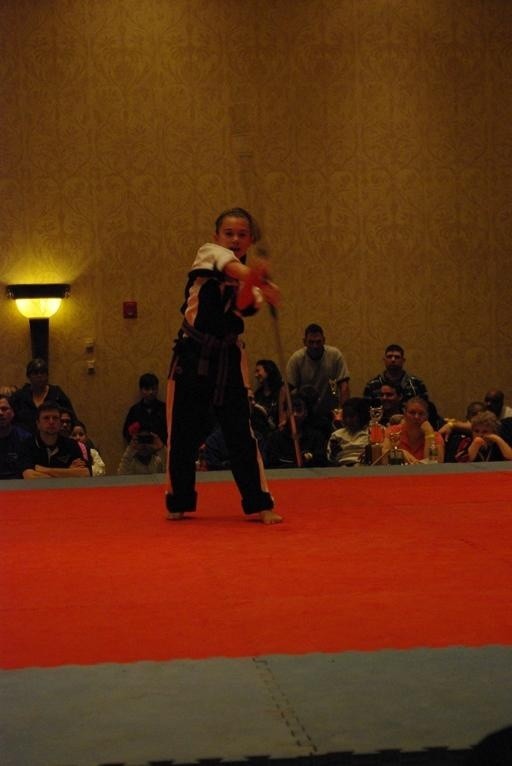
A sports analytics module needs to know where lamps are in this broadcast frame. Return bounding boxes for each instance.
[3,282,71,370]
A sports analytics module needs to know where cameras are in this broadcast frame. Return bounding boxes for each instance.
[136,432,155,445]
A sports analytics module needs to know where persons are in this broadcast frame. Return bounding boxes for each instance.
[159,204,284,529]
[0,355,106,476]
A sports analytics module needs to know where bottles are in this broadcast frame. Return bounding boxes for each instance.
[428,434,438,464]
